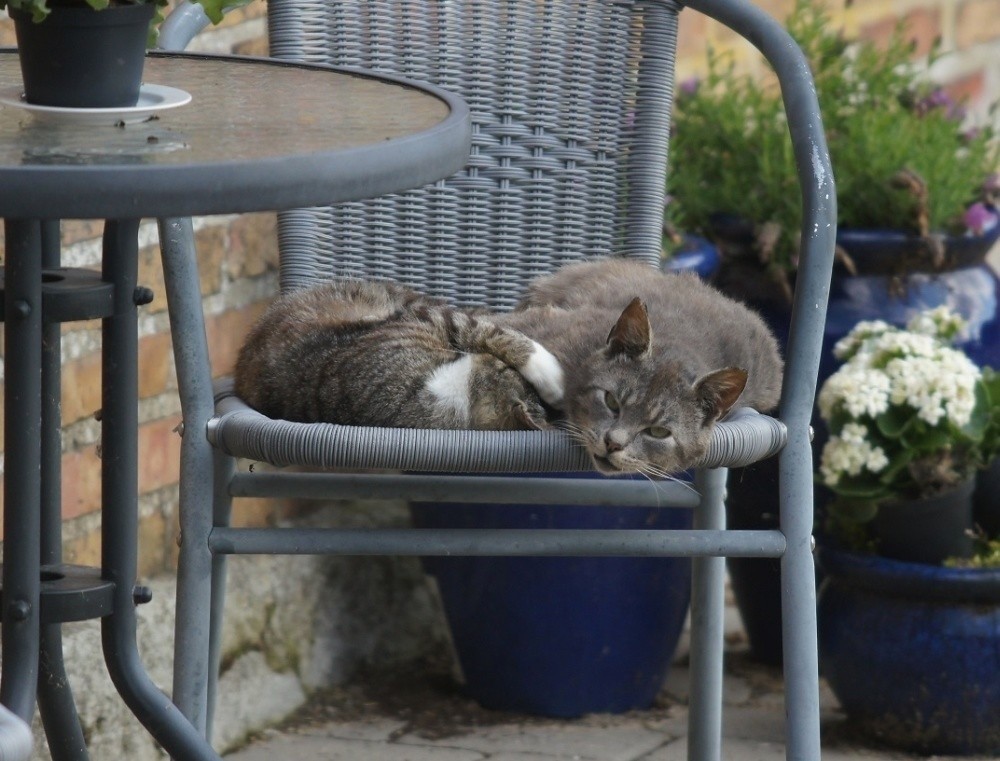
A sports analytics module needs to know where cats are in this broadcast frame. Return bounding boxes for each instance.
[231,254,786,506]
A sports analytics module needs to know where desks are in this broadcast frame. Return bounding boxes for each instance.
[0,47,473,761]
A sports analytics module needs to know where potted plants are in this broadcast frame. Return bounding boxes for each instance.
[403,211,1000,761]
[0,0,254,120]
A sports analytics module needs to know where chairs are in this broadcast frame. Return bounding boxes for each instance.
[154,0,839,761]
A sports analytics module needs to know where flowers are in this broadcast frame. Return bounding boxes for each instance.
[624,0,1000,524]
